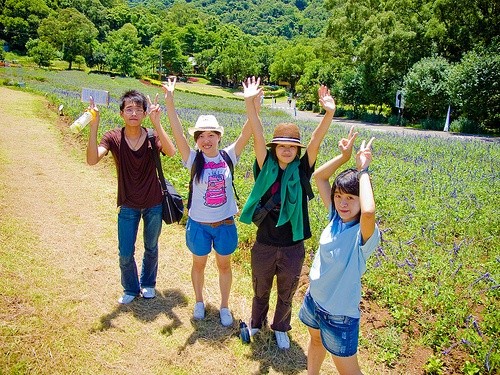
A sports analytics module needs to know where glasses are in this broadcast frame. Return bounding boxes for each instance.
[122,108,144,115]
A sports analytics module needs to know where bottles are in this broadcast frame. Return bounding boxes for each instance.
[70,107,98,133]
[238,319,251,346]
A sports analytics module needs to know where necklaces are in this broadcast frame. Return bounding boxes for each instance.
[204,153,221,178]
[124,127,142,151]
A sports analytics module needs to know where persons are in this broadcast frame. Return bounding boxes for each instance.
[87,89,177,304]
[298,126,380,375]
[161,76,264,326]
[233,76,335,349]
[287,97,291,107]
[294,108,297,116]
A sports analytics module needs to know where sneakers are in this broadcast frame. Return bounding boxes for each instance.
[275,330,290,349]
[194,302,204,319]
[251,327,259,336]
[219,307,233,326]
[118,292,135,304]
[140,285,155,298]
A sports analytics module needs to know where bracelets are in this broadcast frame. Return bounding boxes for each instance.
[356,166,374,182]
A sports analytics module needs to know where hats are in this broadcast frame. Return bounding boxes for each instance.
[188,115,224,137]
[265,122,307,148]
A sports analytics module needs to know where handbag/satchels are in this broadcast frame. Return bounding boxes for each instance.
[159,178,184,225]
